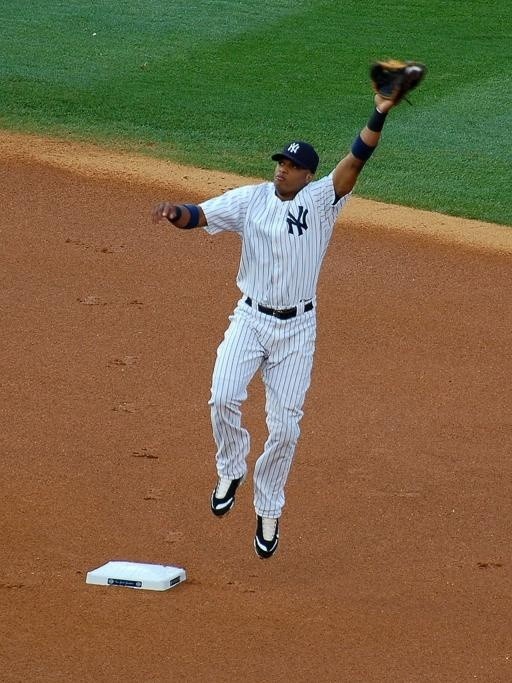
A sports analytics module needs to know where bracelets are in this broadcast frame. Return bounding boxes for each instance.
[367,108,388,133]
[168,206,182,224]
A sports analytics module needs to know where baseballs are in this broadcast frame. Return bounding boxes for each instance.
[406,65,420,76]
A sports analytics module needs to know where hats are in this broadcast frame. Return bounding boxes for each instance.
[271,140,319,173]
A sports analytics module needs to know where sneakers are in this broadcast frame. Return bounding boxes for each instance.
[210,467,247,515]
[253,515,280,559]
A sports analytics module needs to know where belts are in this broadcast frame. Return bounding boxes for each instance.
[245,296,313,319]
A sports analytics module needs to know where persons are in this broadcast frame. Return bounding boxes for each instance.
[149,80,409,560]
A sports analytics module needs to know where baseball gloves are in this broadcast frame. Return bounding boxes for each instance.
[369,58,428,103]
